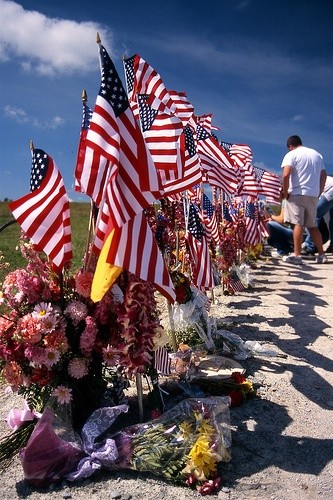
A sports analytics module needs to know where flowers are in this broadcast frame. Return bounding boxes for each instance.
[1,269,254,496]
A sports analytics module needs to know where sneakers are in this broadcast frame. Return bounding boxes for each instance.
[316,254,328,263]
[271,251,289,259]
[282,254,302,264]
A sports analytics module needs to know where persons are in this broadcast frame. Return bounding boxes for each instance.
[280,135,329,266]
[266,190,306,256]
[297,174,333,260]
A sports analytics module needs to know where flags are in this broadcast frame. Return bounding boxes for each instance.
[71,33,281,310]
[5,148,73,270]
